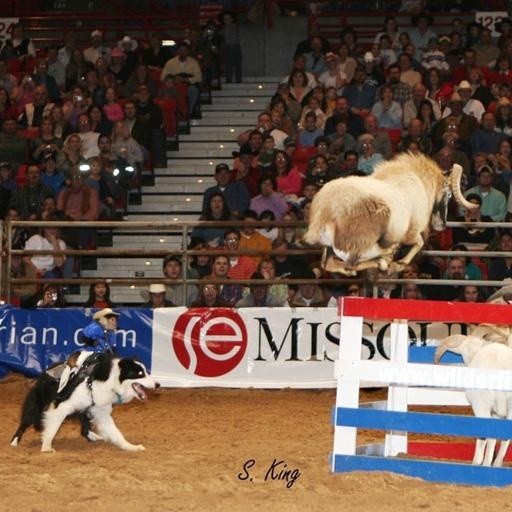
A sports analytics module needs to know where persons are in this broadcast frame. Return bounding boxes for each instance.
[0,0,512,307]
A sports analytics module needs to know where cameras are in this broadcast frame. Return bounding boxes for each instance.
[74,96,85,102]
[27,77,33,82]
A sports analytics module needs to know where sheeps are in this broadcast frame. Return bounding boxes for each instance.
[434,323,512,467]
[300,149,480,277]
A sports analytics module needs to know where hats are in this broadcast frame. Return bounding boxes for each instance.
[447,93,466,107]
[322,52,338,64]
[118,36,138,51]
[140,283,175,301]
[216,163,228,172]
[232,144,259,156]
[477,164,494,175]
[92,308,121,320]
[91,30,102,36]
[454,79,480,97]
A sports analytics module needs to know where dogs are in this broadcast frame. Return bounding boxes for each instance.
[9,351,161,452]
